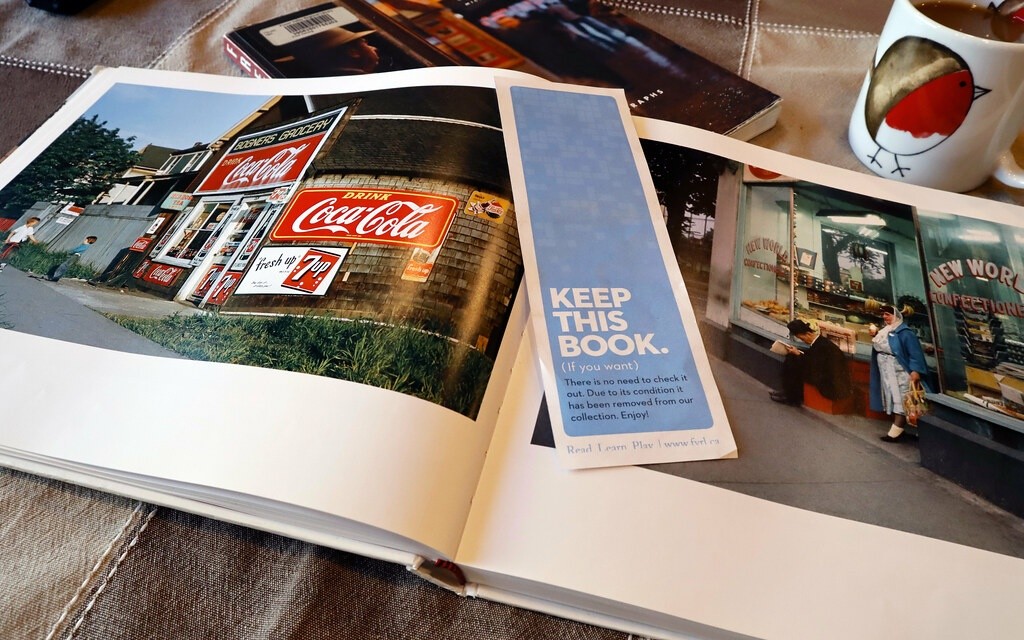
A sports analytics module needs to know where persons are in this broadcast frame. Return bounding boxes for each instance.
[771,319,849,409]
[868,306,932,444]
[48,236,97,282]
[0,217,41,273]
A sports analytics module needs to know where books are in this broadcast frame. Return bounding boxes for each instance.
[0,50,1024,639]
[441,0,784,144]
[224,0,564,84]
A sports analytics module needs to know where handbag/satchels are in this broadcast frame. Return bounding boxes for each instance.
[900,379,936,428]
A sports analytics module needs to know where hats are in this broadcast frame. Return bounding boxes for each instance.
[787,319,811,336]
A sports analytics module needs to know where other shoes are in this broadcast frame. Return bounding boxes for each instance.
[45,277,59,282]
[768,391,785,396]
[880,430,908,443]
[771,396,801,407]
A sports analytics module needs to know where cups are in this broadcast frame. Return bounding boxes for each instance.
[848,0,1024,193]
